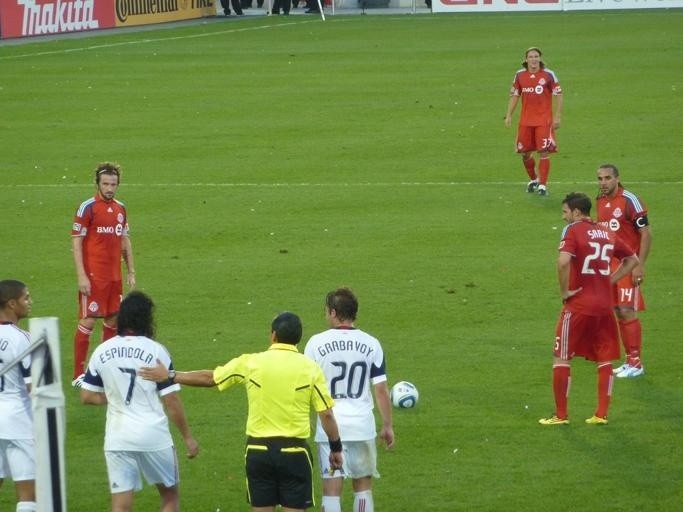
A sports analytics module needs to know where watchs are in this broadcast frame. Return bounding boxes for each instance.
[168,370,176,386]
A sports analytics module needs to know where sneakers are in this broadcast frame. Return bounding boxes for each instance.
[537,184,547,195]
[538,414,570,425]
[616,364,644,378]
[612,363,629,374]
[527,179,539,193]
[585,415,608,425]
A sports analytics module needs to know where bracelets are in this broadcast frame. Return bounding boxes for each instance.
[328,439,343,453]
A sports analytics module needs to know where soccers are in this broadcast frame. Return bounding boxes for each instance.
[392,381,418,409]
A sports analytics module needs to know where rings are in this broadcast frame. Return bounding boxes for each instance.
[638,279,642,281]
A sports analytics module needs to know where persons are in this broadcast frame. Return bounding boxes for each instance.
[596,165,652,379]
[0,280,35,512]
[80,291,199,512]
[220,1,321,16]
[140,313,343,512]
[506,47,563,194]
[539,194,640,426]
[303,288,394,512]
[72,162,136,389]
